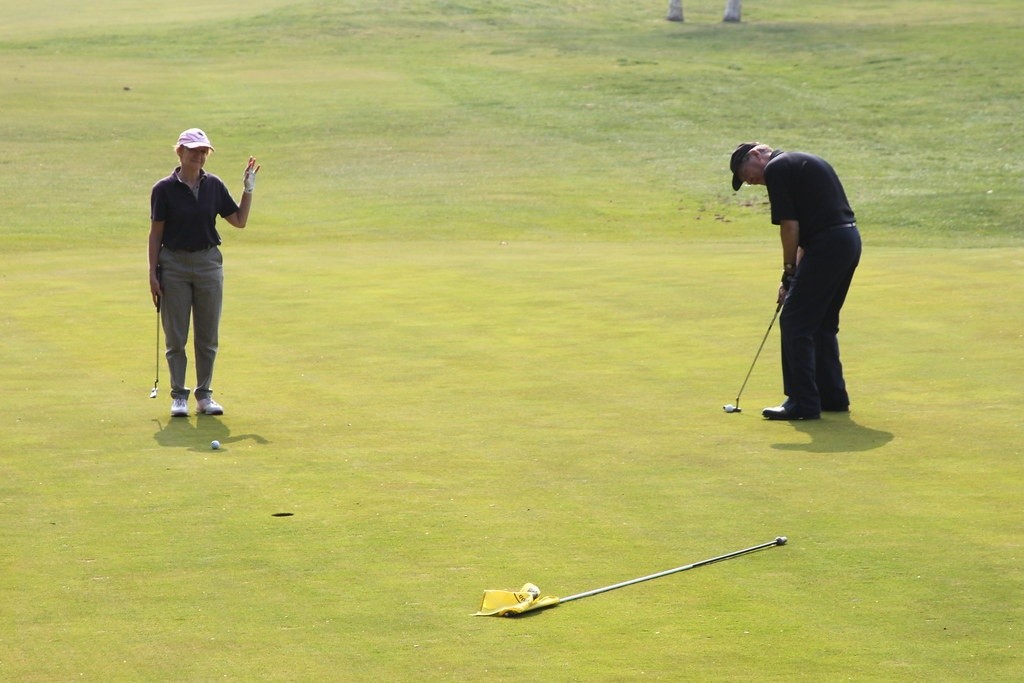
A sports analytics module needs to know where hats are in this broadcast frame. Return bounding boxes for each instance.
[175,127,214,152]
[729,141,761,190]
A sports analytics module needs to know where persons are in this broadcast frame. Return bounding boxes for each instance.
[730,141,862,419]
[664,0,742,22]
[147,127,261,416]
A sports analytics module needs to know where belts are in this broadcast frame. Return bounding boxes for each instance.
[161,240,214,252]
[824,221,857,230]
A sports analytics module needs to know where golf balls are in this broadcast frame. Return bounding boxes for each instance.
[211,440,220,449]
[725,405,734,413]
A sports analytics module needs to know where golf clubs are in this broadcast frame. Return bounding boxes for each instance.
[149,263,161,398]
[723,303,783,413]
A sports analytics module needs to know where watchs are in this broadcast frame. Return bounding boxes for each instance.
[783,262,797,269]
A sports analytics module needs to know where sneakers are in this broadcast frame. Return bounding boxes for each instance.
[170,398,188,416]
[194,397,223,415]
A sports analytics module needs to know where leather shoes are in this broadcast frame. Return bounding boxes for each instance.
[762,396,821,420]
[821,393,848,411]
